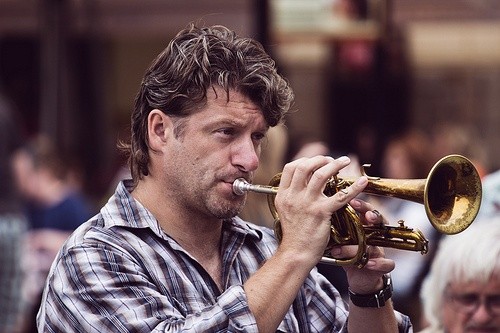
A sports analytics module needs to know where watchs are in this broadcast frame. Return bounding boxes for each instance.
[348,272,394,309]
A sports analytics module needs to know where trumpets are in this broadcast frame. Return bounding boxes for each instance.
[232,153,483,271]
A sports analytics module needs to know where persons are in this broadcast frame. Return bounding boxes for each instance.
[317,1,406,179]
[412,216,499,333]
[238,111,499,315]
[35,18,413,333]
[14,129,100,333]
